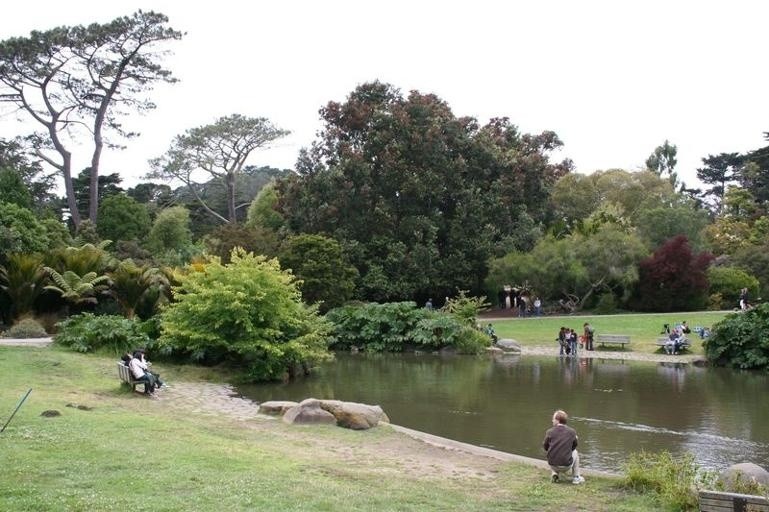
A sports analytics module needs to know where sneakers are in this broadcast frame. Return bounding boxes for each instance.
[551,473,558,484]
[572,476,585,484]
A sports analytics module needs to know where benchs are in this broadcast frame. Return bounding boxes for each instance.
[596,334,630,349]
[117,363,144,393]
[656,338,692,352]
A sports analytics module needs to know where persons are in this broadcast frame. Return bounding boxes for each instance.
[121,352,163,391]
[583,323,594,349]
[487,323,497,345]
[558,326,577,356]
[498,287,541,317]
[425,298,433,308]
[129,352,155,397]
[663,321,691,355]
[541,410,585,485]
